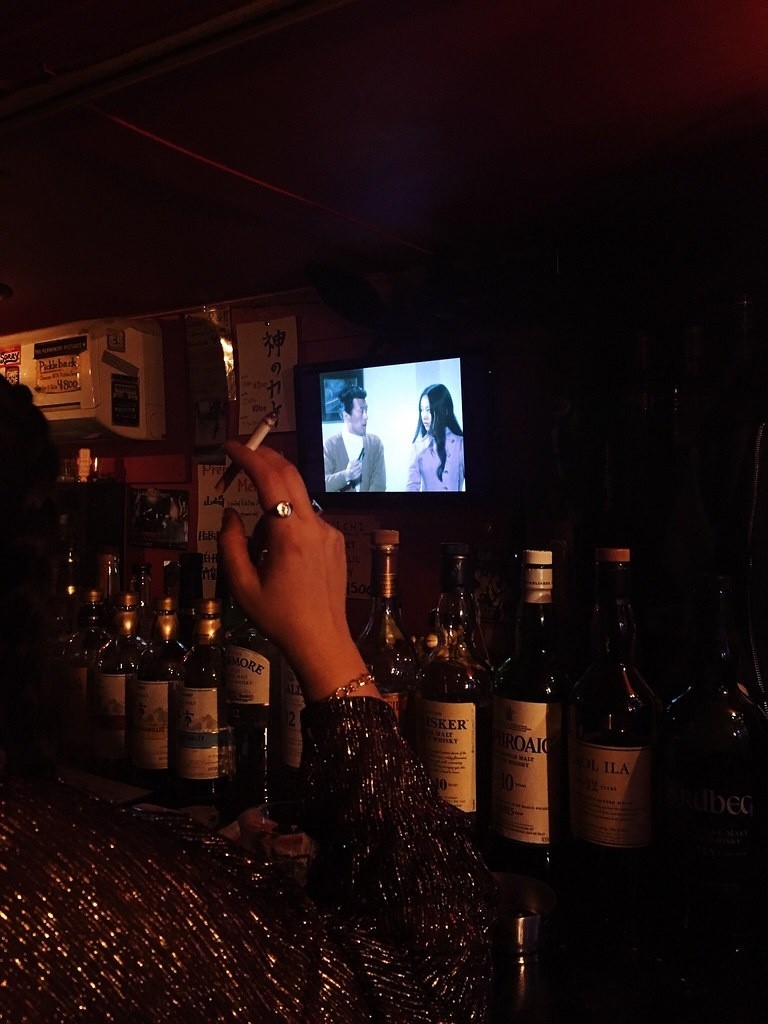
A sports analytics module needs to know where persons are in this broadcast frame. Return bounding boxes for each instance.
[405,383,465,491]
[0,375,505,1024]
[324,385,386,493]
[131,491,188,532]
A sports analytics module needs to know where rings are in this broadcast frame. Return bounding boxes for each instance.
[311,499,322,515]
[266,502,293,518]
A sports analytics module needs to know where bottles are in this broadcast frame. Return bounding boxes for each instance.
[64,531,768,875]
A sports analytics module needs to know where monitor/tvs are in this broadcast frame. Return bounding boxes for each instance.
[321,357,466,493]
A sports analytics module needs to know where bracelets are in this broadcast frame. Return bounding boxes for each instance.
[328,672,374,698]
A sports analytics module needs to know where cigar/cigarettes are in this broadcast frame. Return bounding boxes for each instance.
[214,412,280,496]
